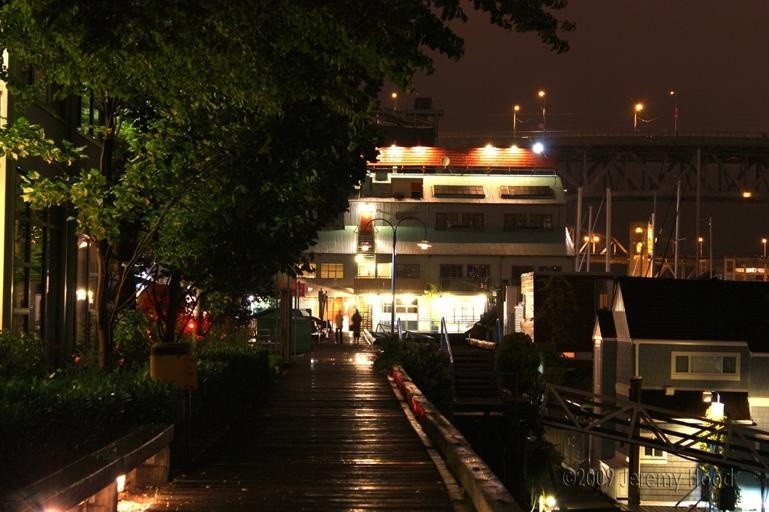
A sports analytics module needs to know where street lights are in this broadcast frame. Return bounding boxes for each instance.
[632,103,645,131]
[538,89,548,140]
[392,92,397,110]
[77,234,91,353]
[358,217,433,339]
[669,91,679,137]
[512,102,521,139]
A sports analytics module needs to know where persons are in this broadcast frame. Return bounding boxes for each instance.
[351,310,362,347]
[335,311,343,345]
